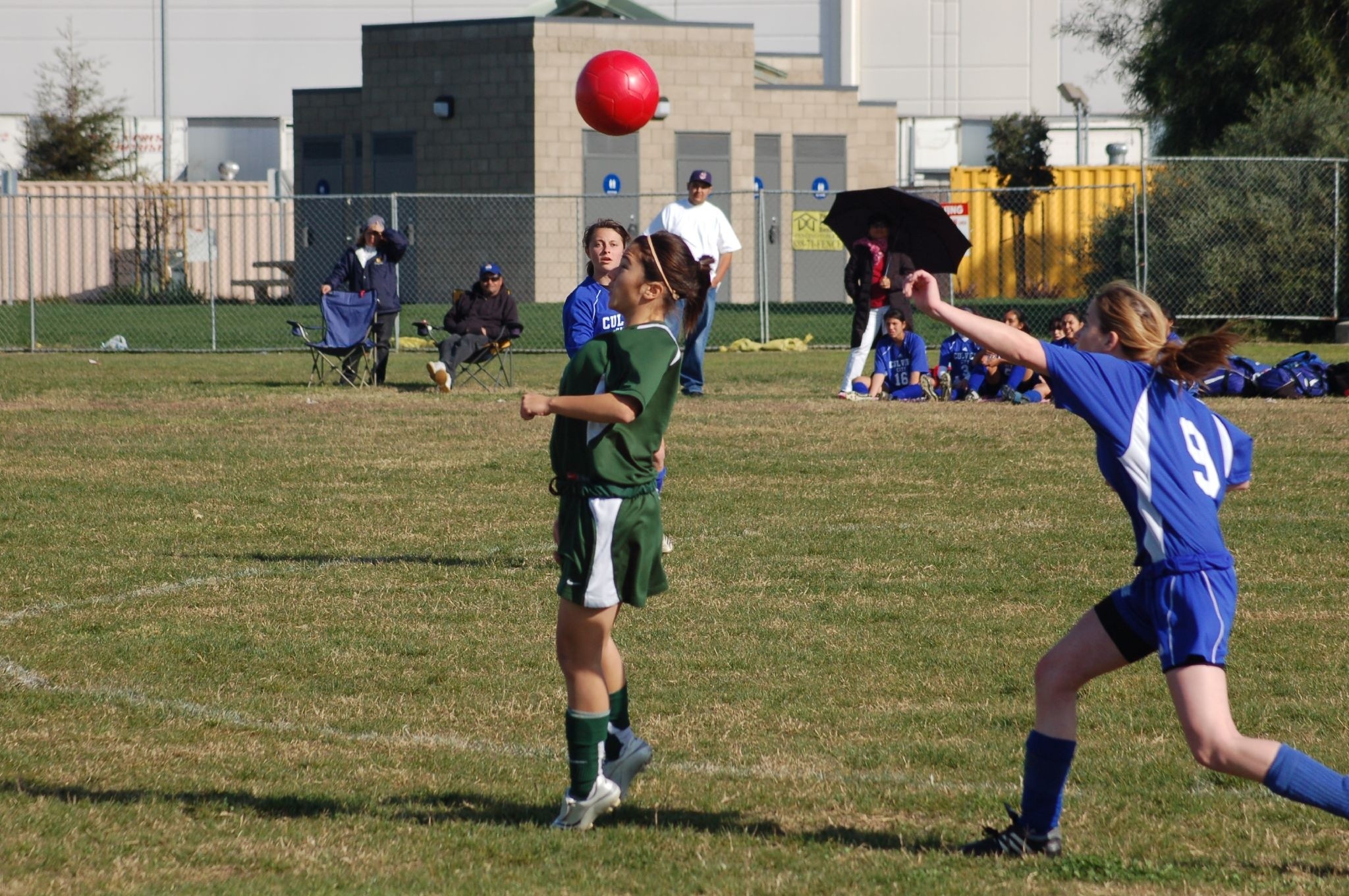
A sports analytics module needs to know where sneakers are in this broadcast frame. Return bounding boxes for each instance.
[604,737,656,796]
[549,773,621,830]
[961,800,1062,858]
[434,369,453,393]
[427,360,447,380]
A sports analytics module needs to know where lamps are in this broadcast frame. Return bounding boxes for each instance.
[651,96,671,120]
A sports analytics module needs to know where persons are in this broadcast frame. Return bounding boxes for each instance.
[648,171,740,397]
[1158,303,1200,397]
[320,214,408,387]
[847,307,930,402]
[426,261,522,393]
[903,269,1349,861]
[520,231,712,829]
[920,304,1084,404]
[837,214,915,401]
[563,220,674,555]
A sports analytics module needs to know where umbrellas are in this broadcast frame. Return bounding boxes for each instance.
[822,185,973,278]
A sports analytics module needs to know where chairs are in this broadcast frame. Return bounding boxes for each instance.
[410,286,516,394]
[286,288,387,391]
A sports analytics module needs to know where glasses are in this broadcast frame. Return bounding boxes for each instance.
[483,277,501,281]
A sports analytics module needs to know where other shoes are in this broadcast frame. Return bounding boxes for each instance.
[1002,386,1017,400]
[963,394,974,401]
[839,391,850,398]
[919,375,940,402]
[689,391,702,398]
[940,373,952,401]
[662,533,676,555]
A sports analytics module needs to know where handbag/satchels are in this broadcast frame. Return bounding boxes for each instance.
[1195,350,1349,399]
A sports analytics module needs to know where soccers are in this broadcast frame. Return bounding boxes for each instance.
[576,49,659,136]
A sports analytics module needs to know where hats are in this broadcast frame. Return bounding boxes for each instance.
[479,263,503,276]
[689,170,712,187]
